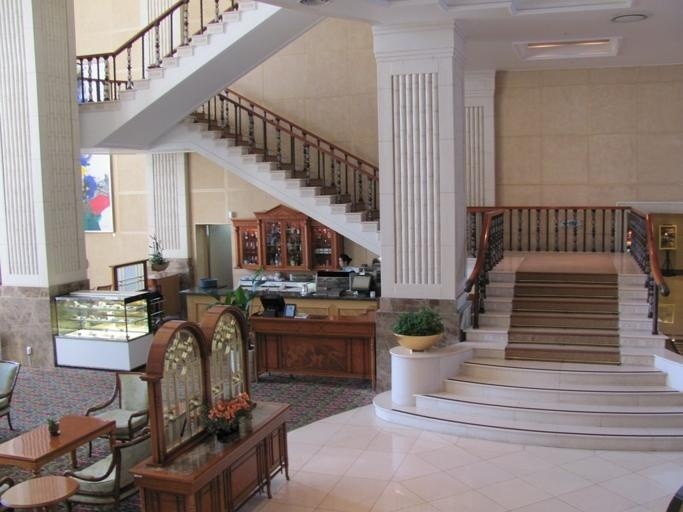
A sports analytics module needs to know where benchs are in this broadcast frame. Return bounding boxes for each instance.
[63,412,186,512]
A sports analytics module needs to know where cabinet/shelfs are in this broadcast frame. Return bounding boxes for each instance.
[308,219,343,270]
[53,296,149,371]
[231,219,259,269]
[258,216,308,271]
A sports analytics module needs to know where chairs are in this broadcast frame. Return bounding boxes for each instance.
[0,477,14,512]
[0,360,21,431]
[85,371,149,457]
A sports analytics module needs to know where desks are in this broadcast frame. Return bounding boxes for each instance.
[129,402,290,512]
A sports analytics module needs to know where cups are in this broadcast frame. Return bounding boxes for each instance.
[299,284,308,296]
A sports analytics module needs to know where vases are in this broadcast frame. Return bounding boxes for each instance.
[216,425,239,443]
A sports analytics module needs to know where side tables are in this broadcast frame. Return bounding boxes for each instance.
[0,475,79,512]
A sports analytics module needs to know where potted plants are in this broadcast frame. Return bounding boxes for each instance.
[46,417,60,435]
[392,308,445,352]
[148,235,170,270]
[206,287,265,382]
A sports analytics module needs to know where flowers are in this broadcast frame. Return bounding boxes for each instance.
[202,393,255,432]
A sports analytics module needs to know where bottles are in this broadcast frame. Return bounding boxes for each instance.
[264,222,281,265]
[313,228,331,267]
[287,226,304,266]
[241,228,257,265]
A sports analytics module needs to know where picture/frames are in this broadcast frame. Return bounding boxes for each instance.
[77,59,115,233]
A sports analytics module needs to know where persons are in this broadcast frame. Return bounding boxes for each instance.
[337,254,354,272]
